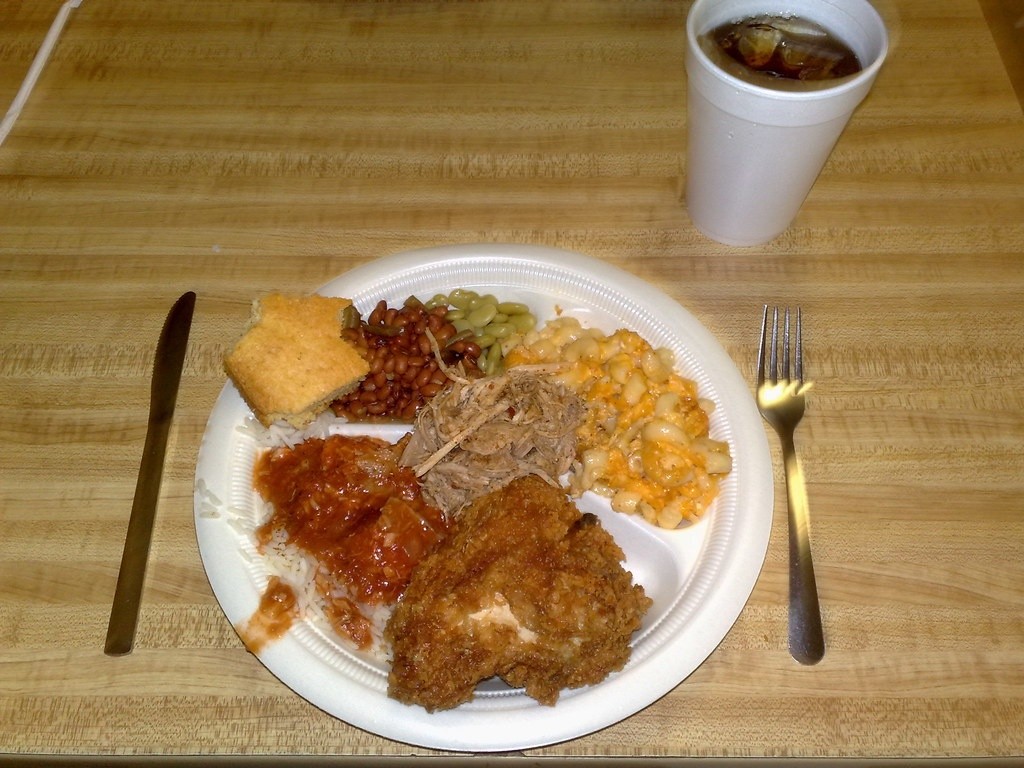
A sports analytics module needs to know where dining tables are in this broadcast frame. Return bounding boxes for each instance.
[0,0,1024,768]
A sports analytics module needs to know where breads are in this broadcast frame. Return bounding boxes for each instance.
[222,294,370,432]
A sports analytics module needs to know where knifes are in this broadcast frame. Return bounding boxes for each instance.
[103,290,195,657]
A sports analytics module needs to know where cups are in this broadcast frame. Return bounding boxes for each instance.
[683,0,889,247]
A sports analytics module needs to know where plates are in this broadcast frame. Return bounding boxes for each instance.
[193,242,773,752]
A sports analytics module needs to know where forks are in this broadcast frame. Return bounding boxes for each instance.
[754,305,824,666]
